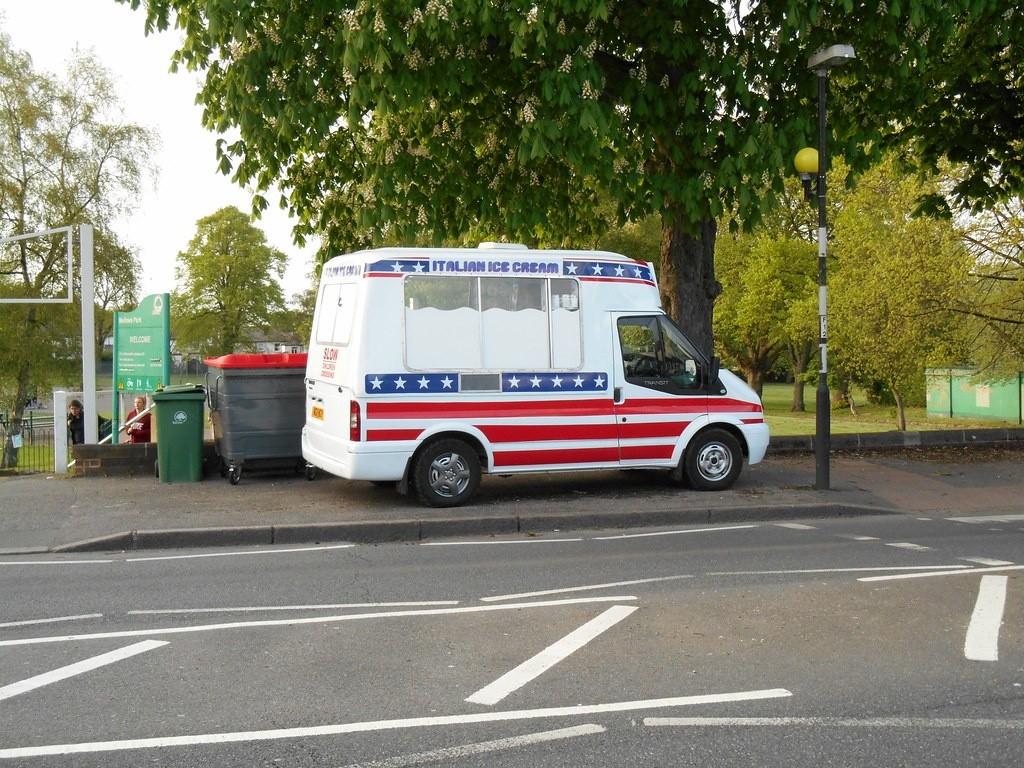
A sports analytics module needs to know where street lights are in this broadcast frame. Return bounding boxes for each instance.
[794,44,857,490]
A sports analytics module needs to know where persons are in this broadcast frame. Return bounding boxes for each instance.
[67,400,105,445]
[126,397,151,443]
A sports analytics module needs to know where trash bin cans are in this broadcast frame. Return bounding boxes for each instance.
[152,382,208,483]
[202,353,318,485]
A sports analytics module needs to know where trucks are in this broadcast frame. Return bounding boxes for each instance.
[302,241,770,508]
